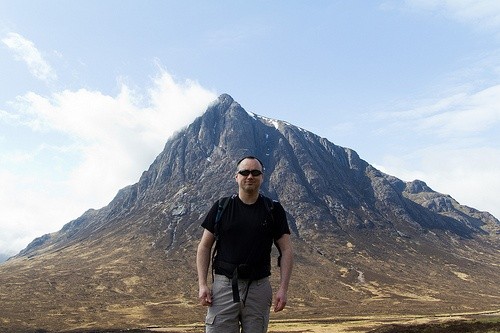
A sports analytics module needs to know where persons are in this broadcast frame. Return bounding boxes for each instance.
[195,155,295,333]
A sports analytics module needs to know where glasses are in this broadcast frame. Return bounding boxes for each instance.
[237,169,264,177]
